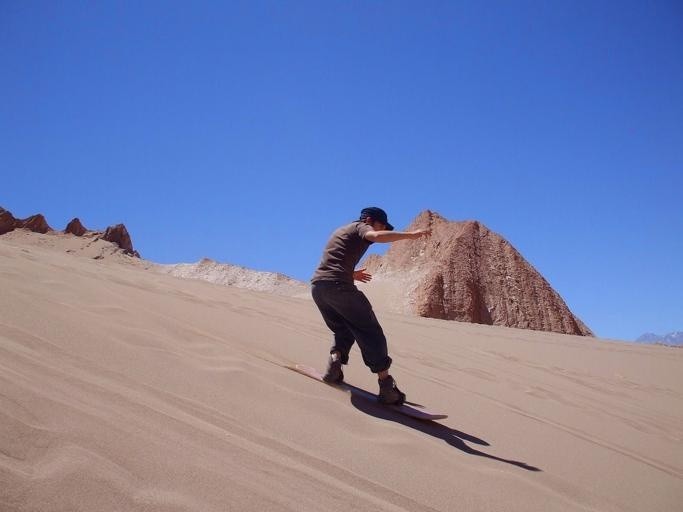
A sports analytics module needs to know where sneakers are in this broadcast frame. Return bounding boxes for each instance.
[378,375,405,404]
[323,358,343,382]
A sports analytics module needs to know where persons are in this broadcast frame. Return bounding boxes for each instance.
[311,208,434,406]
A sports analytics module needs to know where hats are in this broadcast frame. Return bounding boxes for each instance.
[361,208,393,230]
